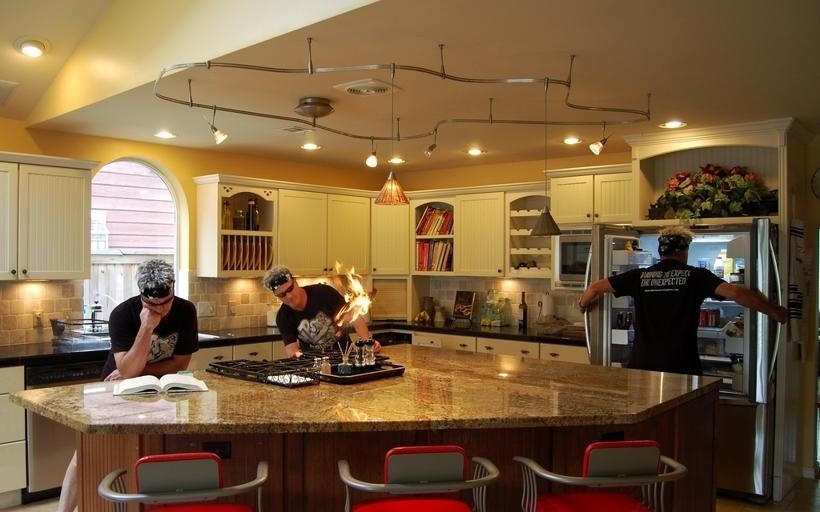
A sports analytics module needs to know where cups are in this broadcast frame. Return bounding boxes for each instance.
[696,256,711,269]
[699,307,721,326]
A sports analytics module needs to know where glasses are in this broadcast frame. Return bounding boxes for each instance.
[270,280,297,299]
[139,282,175,307]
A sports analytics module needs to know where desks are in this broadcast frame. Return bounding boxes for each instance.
[8,344,726,511]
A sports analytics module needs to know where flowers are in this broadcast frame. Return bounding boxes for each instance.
[643,161,777,219]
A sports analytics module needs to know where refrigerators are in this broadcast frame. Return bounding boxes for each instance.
[580,220,782,499]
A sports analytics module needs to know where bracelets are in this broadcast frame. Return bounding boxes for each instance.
[578,296,587,309]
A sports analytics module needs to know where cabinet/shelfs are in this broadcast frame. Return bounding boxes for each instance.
[1,151,104,283]
[452,184,507,277]
[267,335,290,360]
[411,330,438,349]
[441,332,477,356]
[279,180,370,276]
[229,339,275,363]
[478,337,538,362]
[0,365,28,509]
[371,191,429,279]
[538,340,592,365]
[412,186,455,276]
[543,162,635,227]
[179,343,234,369]
[507,179,552,278]
[193,172,279,279]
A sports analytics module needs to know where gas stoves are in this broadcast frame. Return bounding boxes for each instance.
[203,353,390,390]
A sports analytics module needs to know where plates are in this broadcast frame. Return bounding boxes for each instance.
[222,242,274,270]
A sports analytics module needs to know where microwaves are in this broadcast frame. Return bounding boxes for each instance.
[558,235,592,281]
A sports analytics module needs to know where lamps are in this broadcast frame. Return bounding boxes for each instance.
[149,35,656,169]
[373,60,417,206]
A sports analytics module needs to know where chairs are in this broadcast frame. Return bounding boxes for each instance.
[97,451,275,511]
[510,440,689,512]
[323,443,501,511]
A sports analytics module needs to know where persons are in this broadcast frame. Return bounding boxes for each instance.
[262,264,383,359]
[578,226,789,377]
[57,258,200,512]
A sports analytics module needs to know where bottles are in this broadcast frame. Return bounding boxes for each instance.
[313,356,331,382]
[625,238,639,251]
[354,335,376,367]
[518,291,528,330]
[434,321,446,328]
[90,299,103,331]
[434,306,446,321]
[423,296,434,310]
[338,357,353,375]
[480,288,512,328]
[712,248,744,283]
[223,198,260,231]
[519,330,527,336]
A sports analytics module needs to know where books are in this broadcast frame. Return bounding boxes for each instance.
[111,374,209,395]
[415,206,453,271]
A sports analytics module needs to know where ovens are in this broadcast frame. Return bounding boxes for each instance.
[25,362,105,497]
